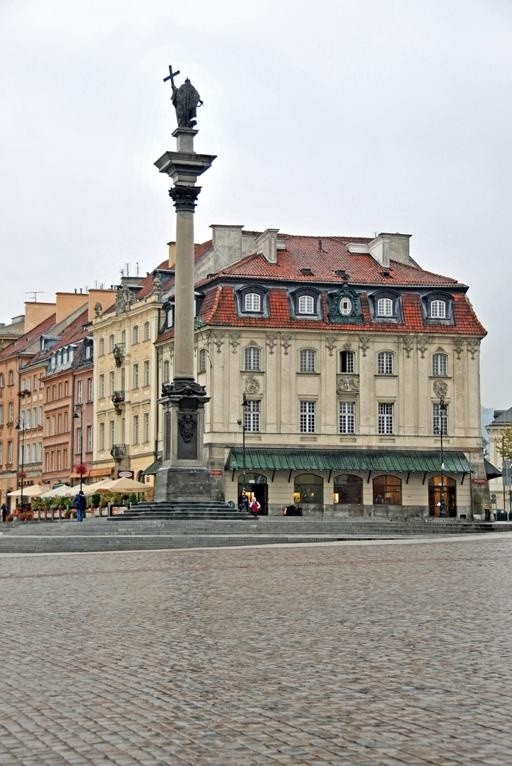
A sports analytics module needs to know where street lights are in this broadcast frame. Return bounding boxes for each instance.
[237,392,249,492]
[72,404,82,491]
[439,399,448,517]
[14,415,24,504]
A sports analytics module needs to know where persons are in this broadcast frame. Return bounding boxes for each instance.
[0,502,9,521]
[239,490,248,511]
[72,489,87,522]
[169,75,202,128]
[249,495,263,516]
[151,272,163,300]
[438,498,447,518]
[448,493,457,517]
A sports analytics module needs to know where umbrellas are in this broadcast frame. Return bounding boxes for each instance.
[57,482,90,497]
[6,482,52,498]
[80,476,114,494]
[39,484,75,497]
[93,476,154,496]
